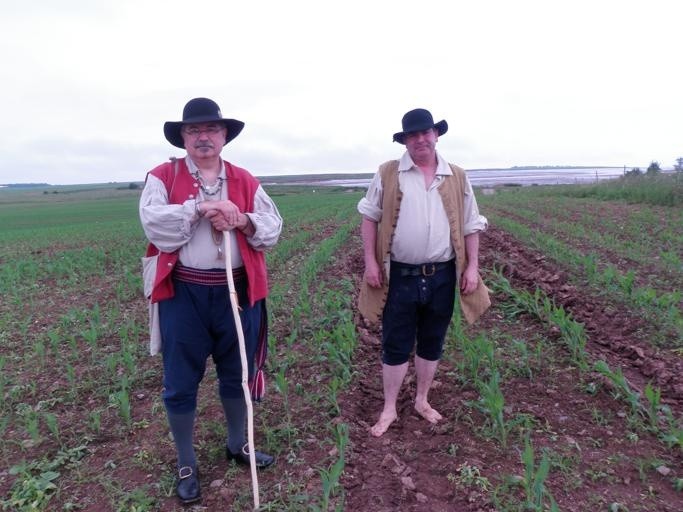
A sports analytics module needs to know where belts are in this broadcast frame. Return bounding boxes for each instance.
[399,261,450,276]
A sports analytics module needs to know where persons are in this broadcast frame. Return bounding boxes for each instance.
[357,109,494,436]
[139,98,284,502]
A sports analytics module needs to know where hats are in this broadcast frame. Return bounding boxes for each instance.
[164,98,244,149]
[394,108,448,144]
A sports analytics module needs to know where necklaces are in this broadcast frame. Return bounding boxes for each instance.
[195,173,223,195]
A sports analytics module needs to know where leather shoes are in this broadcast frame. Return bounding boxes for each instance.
[225,443,273,467]
[178,464,201,502]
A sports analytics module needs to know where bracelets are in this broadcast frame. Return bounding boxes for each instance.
[196,202,203,217]
[241,216,250,231]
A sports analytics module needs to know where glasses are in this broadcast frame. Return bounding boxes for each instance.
[184,128,223,135]
